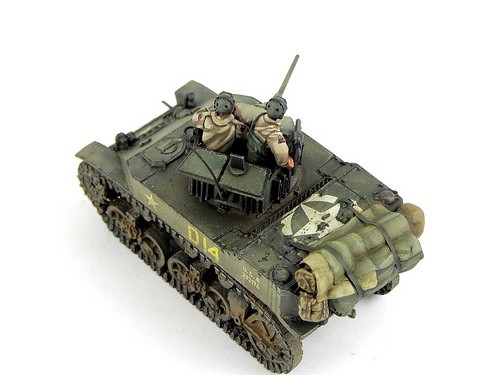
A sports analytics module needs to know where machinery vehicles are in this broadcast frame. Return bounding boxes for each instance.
[76,54,425,371]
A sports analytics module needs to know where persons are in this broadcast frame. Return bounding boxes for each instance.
[192,91,248,154]
[247,95,295,170]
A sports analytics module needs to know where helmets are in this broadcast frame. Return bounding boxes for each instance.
[214,91,236,115]
[266,95,287,120]
